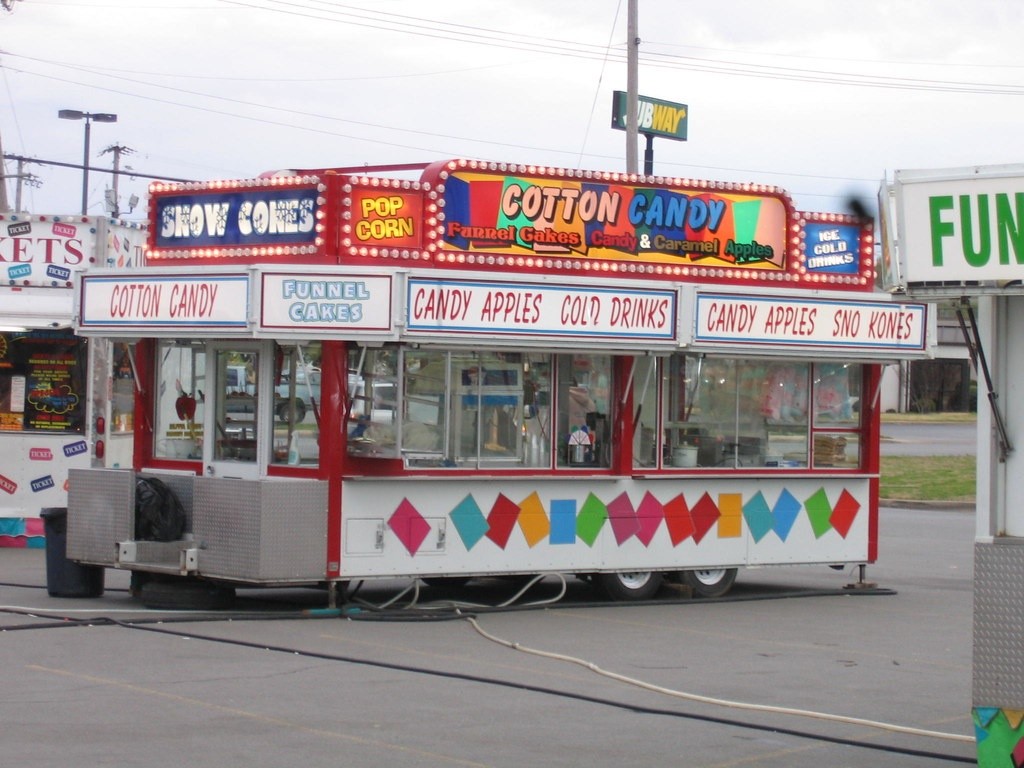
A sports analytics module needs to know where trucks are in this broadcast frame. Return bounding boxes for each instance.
[62,158,938,599]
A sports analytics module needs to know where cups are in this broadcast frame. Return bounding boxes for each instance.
[571,445,584,462]
[522,431,552,467]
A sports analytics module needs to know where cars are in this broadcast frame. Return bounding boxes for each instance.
[347,382,399,421]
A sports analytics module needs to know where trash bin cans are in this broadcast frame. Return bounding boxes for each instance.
[40,506,105,599]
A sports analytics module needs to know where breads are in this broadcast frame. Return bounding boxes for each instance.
[814,434,848,464]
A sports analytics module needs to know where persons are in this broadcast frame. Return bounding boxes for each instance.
[524,372,534,403]
[569,378,594,428]
[348,385,380,436]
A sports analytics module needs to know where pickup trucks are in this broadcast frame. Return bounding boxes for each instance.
[224,362,323,426]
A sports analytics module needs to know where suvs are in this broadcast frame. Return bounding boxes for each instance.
[283,368,321,385]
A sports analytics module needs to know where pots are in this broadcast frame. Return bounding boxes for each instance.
[671,441,699,467]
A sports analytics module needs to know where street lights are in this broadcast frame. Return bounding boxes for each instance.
[58,110,118,216]
